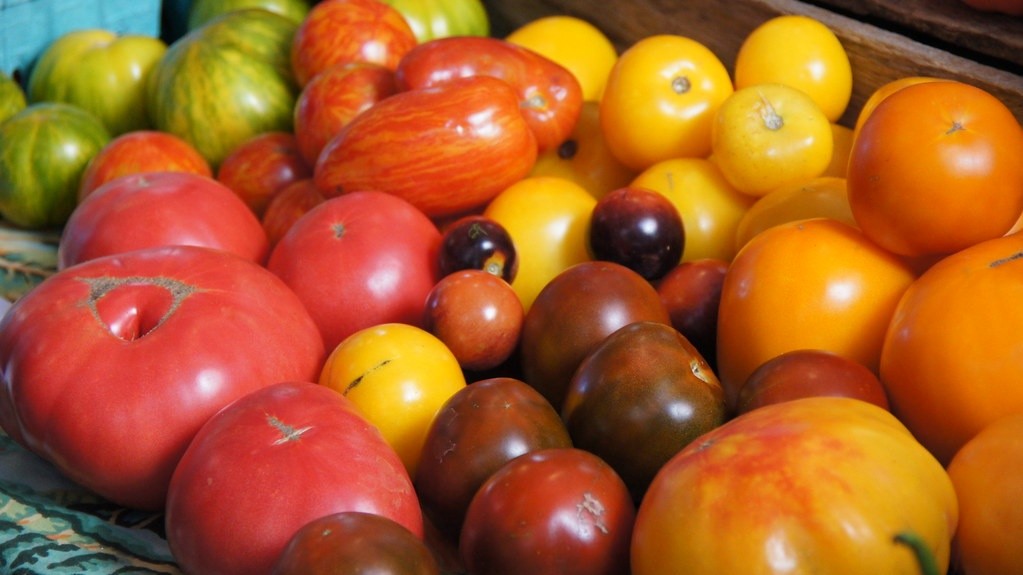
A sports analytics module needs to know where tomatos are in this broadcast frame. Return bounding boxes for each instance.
[0,0,1023,575]
